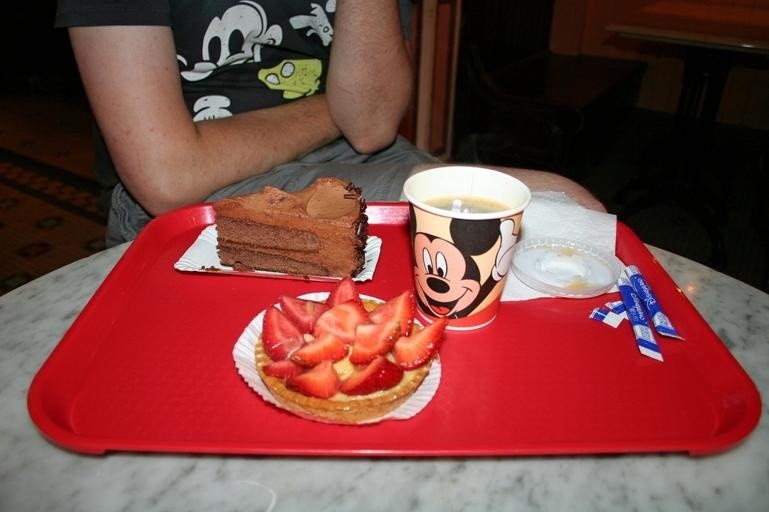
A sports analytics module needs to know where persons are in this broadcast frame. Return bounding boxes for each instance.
[55,1,612,240]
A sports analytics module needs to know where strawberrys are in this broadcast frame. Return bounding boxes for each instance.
[261,277,449,399]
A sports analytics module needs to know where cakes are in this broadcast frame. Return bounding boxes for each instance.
[211,175,366,278]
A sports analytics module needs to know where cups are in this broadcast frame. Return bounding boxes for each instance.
[402,165,532,332]
